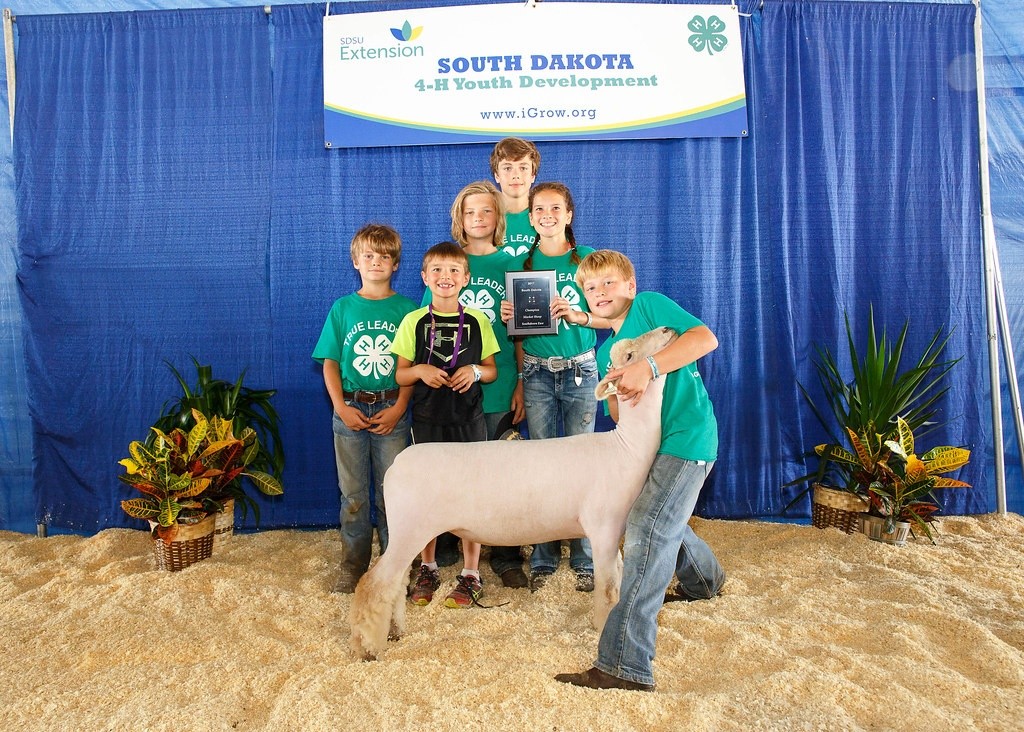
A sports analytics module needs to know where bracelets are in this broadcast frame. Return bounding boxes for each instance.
[646,356,660,380]
[582,312,592,328]
[518,373,523,379]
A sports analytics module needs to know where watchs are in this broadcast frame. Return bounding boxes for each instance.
[469,364,482,382]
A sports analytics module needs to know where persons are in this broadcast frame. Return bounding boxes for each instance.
[389,243,501,609]
[312,225,418,595]
[554,251,727,692]
[489,138,542,265]
[499,183,611,590]
[420,180,527,587]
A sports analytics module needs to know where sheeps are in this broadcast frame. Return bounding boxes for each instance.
[349,326,679,662]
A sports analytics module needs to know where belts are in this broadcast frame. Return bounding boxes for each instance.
[523,348,595,373]
[343,390,401,405]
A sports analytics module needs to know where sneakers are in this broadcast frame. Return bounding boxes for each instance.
[411,565,440,605]
[445,574,511,610]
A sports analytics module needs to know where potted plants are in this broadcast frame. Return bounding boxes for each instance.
[141,354,286,551]
[117,408,283,573]
[815,417,971,545]
[782,298,964,535]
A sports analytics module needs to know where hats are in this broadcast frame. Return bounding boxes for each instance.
[493,412,526,442]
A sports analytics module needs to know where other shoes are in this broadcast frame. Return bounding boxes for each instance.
[578,573,595,592]
[555,666,652,693]
[530,568,550,592]
[502,568,528,589]
[663,583,692,603]
[436,544,458,567]
[334,569,359,595]
